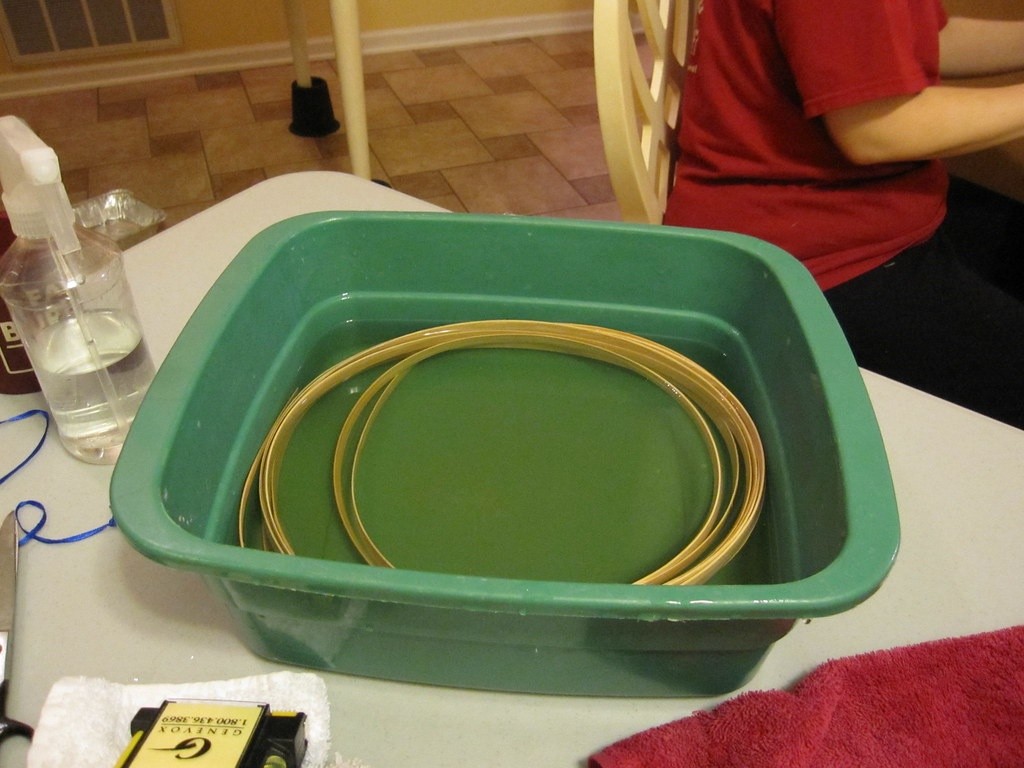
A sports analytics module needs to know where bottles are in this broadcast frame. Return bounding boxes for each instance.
[0,115,157,466]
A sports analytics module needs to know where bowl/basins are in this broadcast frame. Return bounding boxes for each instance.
[113,211,903,698]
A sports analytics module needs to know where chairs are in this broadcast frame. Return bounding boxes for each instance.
[594,0,690,223]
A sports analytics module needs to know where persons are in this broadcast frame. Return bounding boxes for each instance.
[663,0,1024,429]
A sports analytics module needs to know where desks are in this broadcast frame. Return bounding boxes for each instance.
[1,171,1023,768]
